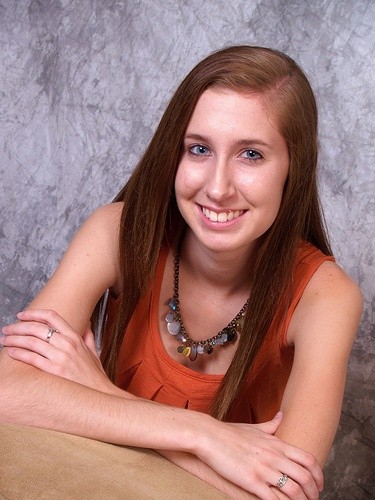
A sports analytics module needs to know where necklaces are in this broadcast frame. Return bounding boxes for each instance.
[164,217,276,357]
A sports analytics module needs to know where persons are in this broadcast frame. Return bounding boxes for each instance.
[1,45,364,500]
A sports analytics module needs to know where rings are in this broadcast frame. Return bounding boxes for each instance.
[45,327,58,340]
[277,474,289,490]
